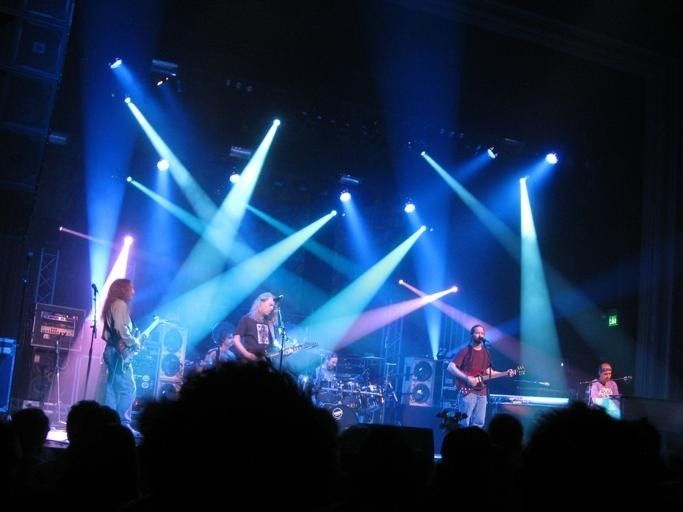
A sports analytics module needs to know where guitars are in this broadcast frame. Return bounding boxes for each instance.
[456,365,525,396]
[103,315,169,364]
[241,344,319,364]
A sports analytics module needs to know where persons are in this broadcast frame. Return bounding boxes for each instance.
[311,352,359,432]
[0,356,682,512]
[233,291,294,367]
[196,320,238,373]
[446,325,515,429]
[98,278,145,439]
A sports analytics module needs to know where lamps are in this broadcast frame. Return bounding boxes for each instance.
[488,145,499,159]
[108,55,122,69]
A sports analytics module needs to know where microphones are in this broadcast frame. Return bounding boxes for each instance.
[480,336,489,343]
[538,381,550,387]
[273,295,282,300]
[623,376,632,381]
[91,283,96,292]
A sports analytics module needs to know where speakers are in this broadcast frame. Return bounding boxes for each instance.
[153,382,183,400]
[0,337,16,412]
[151,325,187,382]
[401,357,436,408]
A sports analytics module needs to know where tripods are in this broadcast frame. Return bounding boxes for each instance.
[44,334,69,423]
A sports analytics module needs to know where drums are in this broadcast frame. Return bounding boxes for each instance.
[323,402,359,430]
[361,386,383,413]
[343,382,362,409]
[317,380,342,403]
